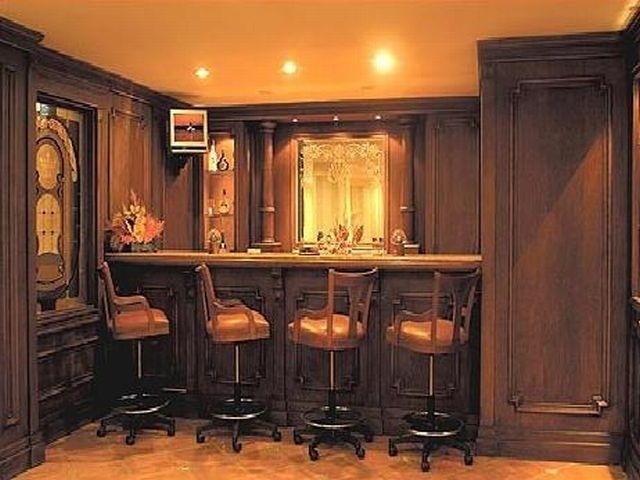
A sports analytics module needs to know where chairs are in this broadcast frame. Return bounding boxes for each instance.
[289,268,386,458]
[194,260,282,452]
[385,267,482,472]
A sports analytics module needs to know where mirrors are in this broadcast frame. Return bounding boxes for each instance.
[289,132,389,252]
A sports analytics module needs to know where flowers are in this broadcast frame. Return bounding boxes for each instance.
[309,225,362,249]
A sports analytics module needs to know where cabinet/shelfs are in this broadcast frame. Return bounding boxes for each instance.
[1,16,193,479]
[192,97,480,254]
[476,31,638,479]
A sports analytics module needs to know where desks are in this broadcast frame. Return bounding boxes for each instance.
[104,250,482,436]
